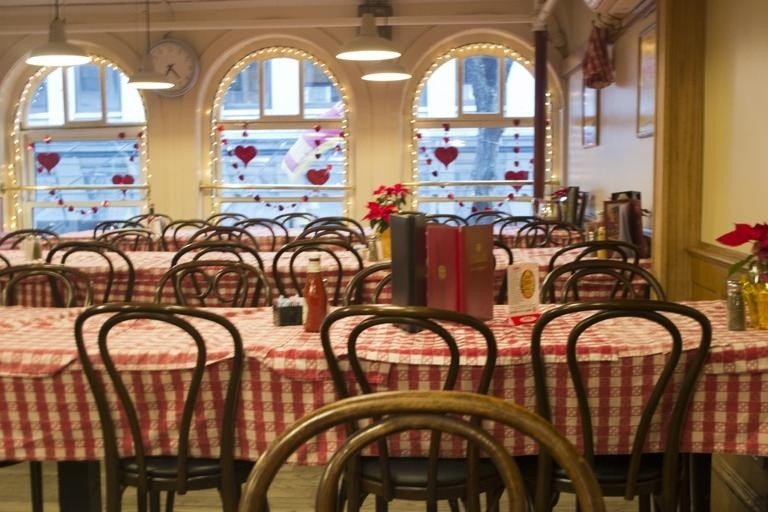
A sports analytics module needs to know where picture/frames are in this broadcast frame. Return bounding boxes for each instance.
[636,22,658,139]
[581,85,600,149]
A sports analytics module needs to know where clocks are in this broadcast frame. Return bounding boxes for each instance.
[150,38,200,97]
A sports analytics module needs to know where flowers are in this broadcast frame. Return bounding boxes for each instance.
[360,185,412,234]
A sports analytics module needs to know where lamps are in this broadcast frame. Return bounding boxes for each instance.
[124,0,174,89]
[335,0,404,61]
[24,0,95,66]
[361,9,412,81]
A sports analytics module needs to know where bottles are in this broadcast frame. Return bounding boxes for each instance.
[27,234,42,260]
[726,280,744,331]
[301,251,326,332]
[584,221,606,258]
[367,235,385,261]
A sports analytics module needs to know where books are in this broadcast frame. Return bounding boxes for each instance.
[387,209,495,333]
